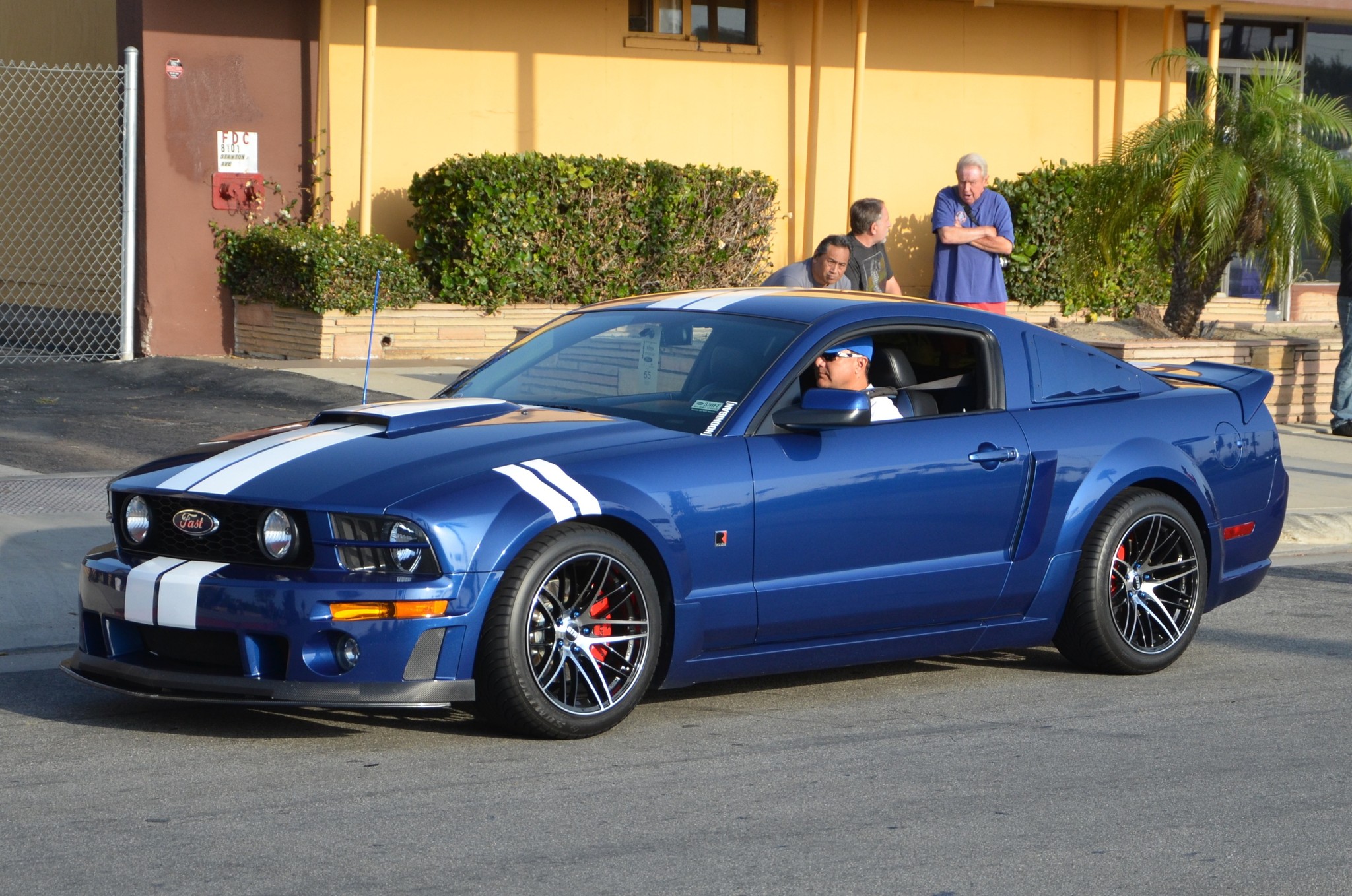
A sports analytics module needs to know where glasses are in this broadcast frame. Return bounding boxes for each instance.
[822,351,864,362]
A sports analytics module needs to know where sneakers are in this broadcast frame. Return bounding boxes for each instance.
[1332,422,1352,437]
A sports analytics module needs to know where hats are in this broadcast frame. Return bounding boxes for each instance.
[824,335,873,362]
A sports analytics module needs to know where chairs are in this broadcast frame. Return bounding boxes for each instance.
[868,345,940,418]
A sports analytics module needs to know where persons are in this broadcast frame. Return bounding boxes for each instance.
[842,198,903,296]
[1329,202,1352,437]
[814,335,905,421]
[928,152,1015,317]
[760,233,854,290]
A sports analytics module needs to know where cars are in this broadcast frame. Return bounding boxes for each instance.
[1228,251,1267,296]
[56,287,1290,738]
